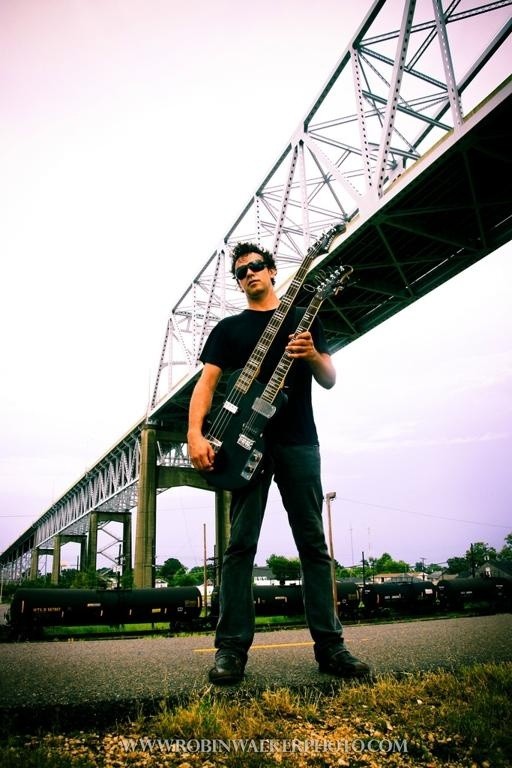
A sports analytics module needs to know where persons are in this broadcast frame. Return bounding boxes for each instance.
[186,239,370,686]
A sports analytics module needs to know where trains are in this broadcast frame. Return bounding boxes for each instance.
[5,575,511,633]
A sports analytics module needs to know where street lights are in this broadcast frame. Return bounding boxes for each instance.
[324,492,339,613]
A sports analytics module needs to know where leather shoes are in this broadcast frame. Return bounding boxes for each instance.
[209,656,243,684]
[319,650,369,677]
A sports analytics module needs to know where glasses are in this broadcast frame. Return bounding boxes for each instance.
[233,258,267,280]
